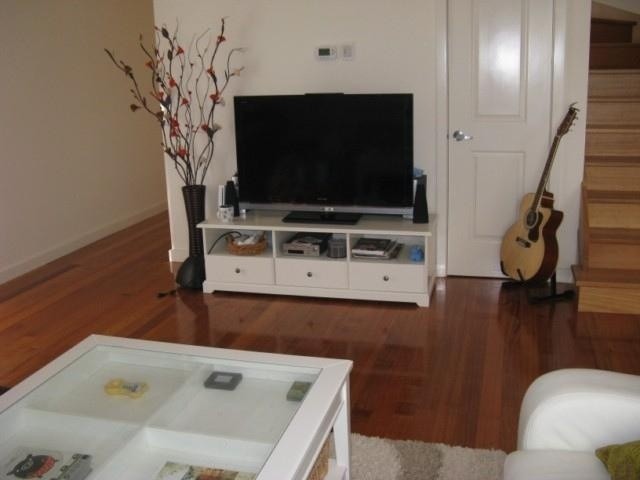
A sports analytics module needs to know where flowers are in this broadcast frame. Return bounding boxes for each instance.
[103,12,246,186]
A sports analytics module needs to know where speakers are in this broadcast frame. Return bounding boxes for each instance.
[231,173,249,213]
[403,174,429,220]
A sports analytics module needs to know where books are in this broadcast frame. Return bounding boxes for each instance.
[352,237,404,261]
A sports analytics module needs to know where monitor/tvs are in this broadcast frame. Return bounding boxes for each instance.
[234,96,414,224]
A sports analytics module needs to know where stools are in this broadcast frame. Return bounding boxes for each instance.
[507,365,640,480]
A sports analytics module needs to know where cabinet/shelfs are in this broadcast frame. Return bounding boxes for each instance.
[191,210,438,309]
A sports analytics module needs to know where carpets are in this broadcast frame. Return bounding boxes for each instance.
[344,432,506,480]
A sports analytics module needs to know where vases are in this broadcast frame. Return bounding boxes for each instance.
[175,188,209,291]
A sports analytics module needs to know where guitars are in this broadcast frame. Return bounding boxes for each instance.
[499,103,579,282]
